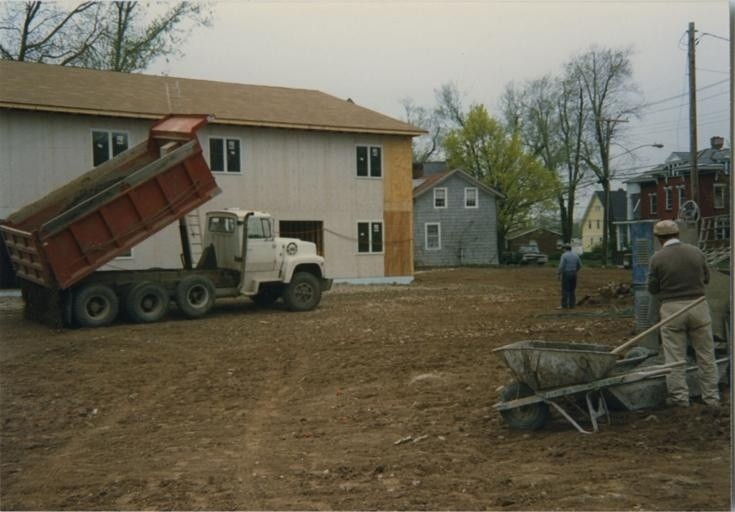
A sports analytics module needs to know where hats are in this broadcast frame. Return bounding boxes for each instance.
[653,220,680,235]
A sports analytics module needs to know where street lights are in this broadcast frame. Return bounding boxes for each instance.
[605,142,664,266]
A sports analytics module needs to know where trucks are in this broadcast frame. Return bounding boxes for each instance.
[0,113,336,329]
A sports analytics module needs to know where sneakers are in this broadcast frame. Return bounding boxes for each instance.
[664,396,689,407]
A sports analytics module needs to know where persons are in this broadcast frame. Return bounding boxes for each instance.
[645,219,722,409]
[556,243,582,311]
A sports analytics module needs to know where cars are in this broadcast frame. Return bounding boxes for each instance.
[499,243,547,265]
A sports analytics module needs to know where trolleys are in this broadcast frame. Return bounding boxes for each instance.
[491,333,685,436]
